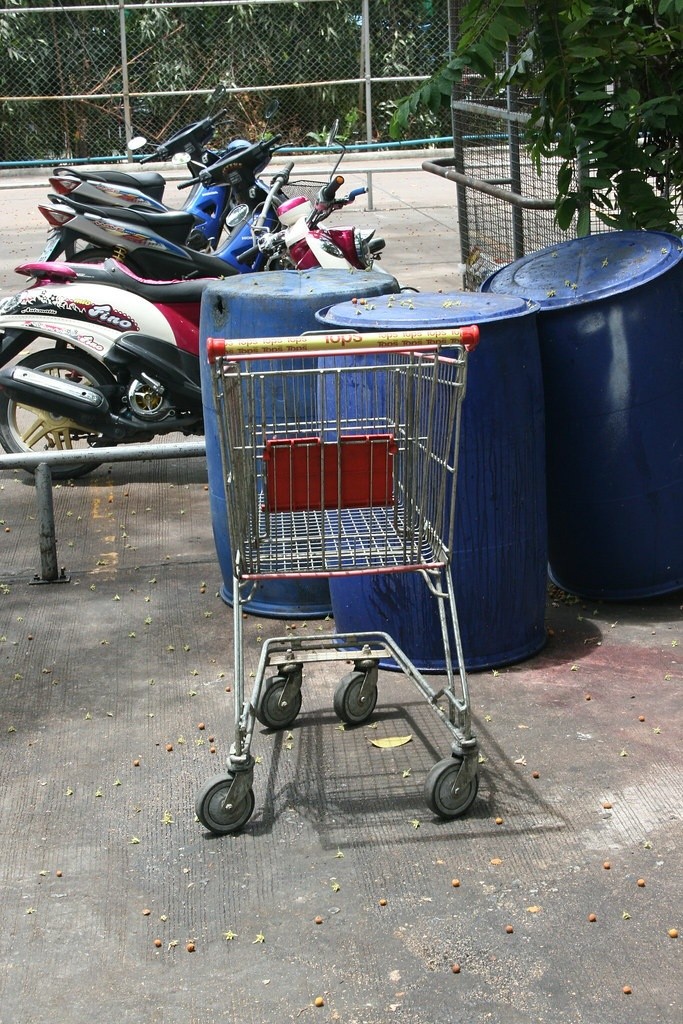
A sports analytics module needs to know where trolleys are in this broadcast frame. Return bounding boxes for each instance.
[196,320,487,846]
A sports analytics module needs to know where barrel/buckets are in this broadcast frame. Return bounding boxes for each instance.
[198,268,401,619]
[482,229,682,602]
[313,291,550,676]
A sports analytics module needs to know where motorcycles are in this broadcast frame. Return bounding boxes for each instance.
[0,86,391,488]
